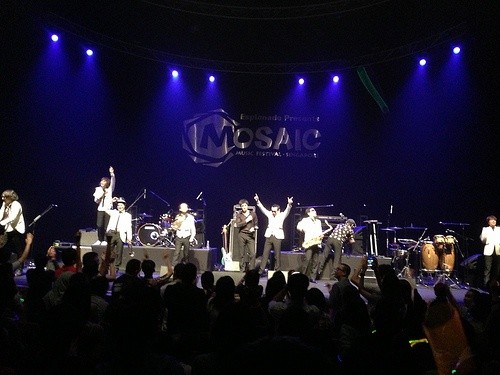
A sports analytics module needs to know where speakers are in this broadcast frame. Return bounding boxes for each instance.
[53,246,75,271]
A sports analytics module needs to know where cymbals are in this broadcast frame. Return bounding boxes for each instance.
[189,208,194,210]
[141,213,154,218]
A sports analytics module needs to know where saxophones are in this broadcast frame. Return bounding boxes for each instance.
[302,220,333,249]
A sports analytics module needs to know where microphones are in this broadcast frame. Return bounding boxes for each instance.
[52,204,58,207]
[196,191,203,200]
[143,188,146,199]
[439,221,445,225]
[390,204,393,214]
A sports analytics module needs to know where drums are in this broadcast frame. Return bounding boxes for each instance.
[445,236,454,244]
[420,240,439,270]
[434,235,444,243]
[437,244,454,270]
[137,224,162,246]
[161,215,174,229]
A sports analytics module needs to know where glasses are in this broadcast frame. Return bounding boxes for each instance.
[272,210,278,213]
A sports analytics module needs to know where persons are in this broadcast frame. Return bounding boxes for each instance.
[236,199,258,273]
[93,166,115,245]
[0,190,25,276]
[104,197,133,276]
[171,203,196,274]
[0,246,500,375]
[318,219,356,280]
[296,207,324,283]
[255,193,293,275]
[480,216,500,290]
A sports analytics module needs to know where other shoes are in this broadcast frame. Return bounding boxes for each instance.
[309,279,317,284]
[16,268,23,275]
[258,271,263,276]
[315,270,319,279]
[330,278,337,281]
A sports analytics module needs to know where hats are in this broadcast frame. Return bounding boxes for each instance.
[347,219,355,224]
[117,197,126,203]
[179,203,189,212]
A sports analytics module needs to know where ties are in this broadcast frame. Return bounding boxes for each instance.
[115,211,122,231]
[102,188,104,207]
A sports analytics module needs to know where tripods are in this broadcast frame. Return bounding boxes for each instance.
[397,229,458,289]
[152,219,177,248]
